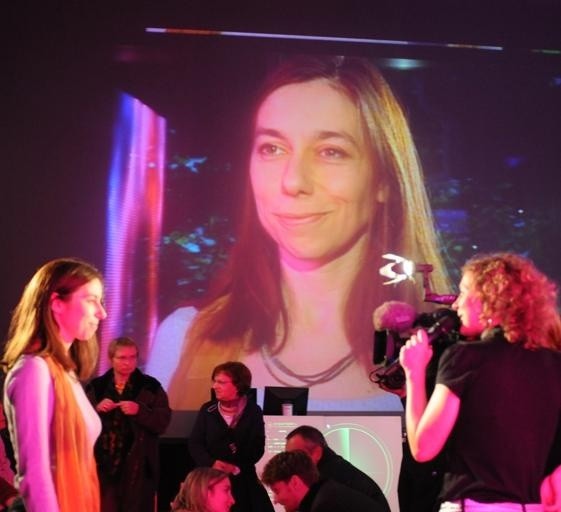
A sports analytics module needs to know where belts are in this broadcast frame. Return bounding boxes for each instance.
[441,500,543,511]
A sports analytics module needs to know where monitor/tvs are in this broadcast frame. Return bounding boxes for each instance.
[211,387,257,405]
[262,385,309,415]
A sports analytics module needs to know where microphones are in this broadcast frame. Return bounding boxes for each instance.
[384,315,455,375]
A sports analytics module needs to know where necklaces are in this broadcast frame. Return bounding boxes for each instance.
[259,340,359,391]
[65,371,81,385]
[114,378,128,391]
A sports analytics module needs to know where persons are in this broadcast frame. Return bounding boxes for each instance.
[284,425,393,511]
[260,446,389,512]
[370,300,463,512]
[1,256,108,512]
[85,335,172,512]
[187,360,277,512]
[1,400,29,511]
[170,466,237,512]
[145,50,459,412]
[397,250,561,512]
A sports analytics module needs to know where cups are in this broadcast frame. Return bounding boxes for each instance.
[282,404,293,416]
